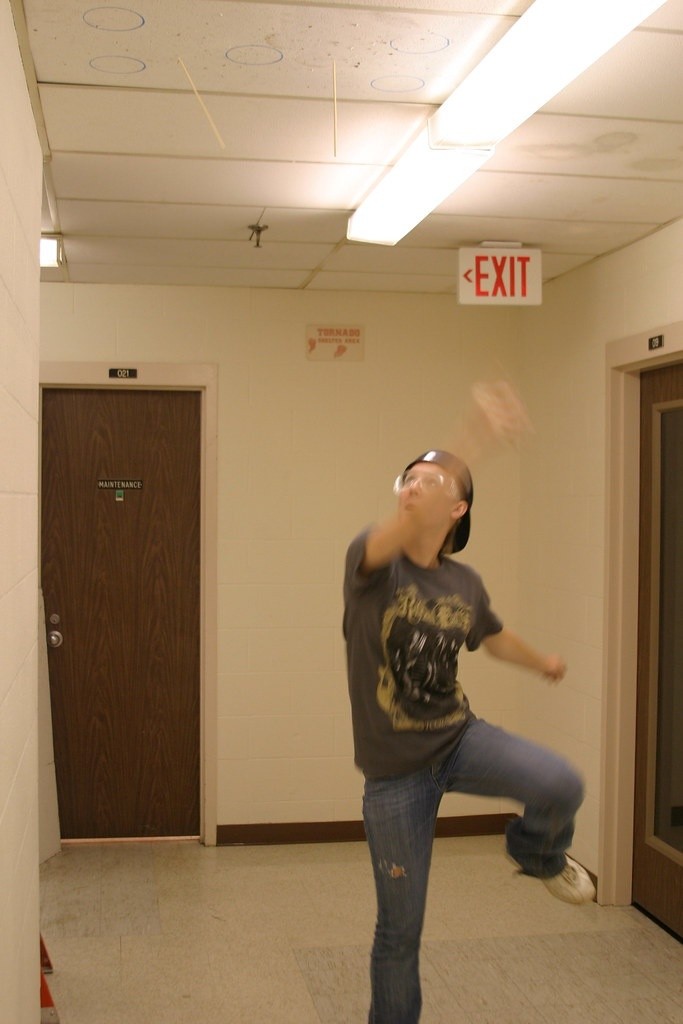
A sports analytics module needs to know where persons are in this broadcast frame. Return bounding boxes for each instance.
[343,452,596,1024]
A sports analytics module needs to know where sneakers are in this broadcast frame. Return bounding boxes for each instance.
[506,851,596,906]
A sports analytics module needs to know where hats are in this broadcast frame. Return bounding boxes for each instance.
[403,449,474,555]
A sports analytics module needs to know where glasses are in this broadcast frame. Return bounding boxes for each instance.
[394,471,446,500]
